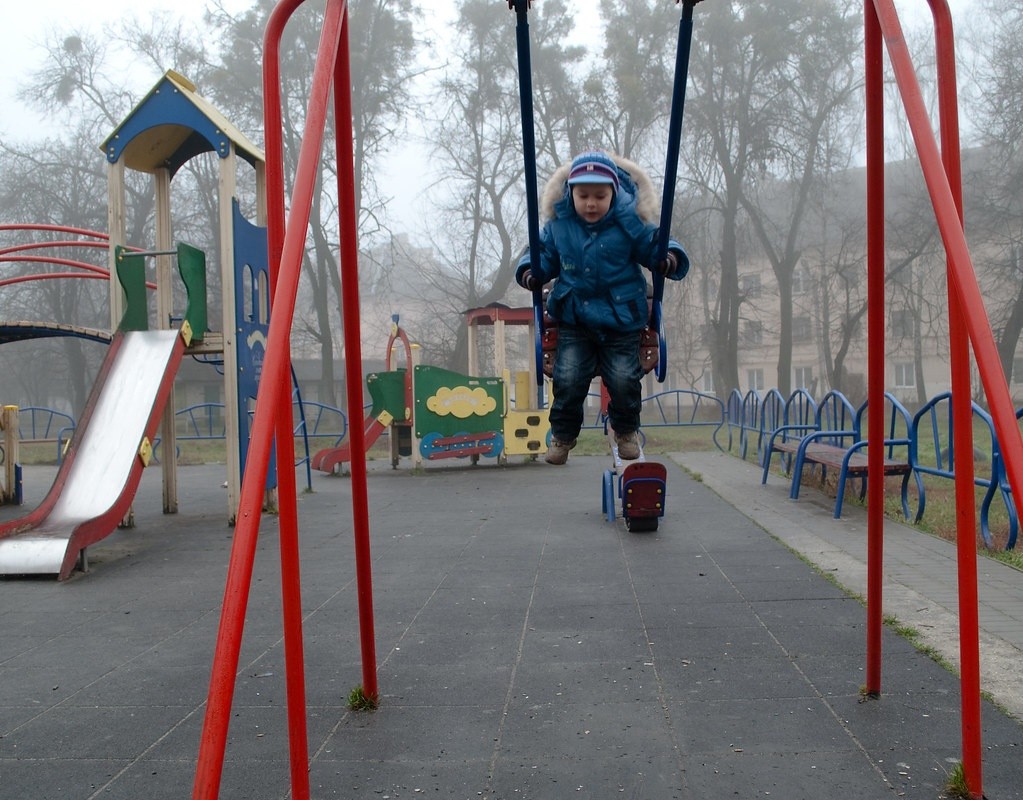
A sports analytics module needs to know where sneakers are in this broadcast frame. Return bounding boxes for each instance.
[545,437,576,465]
[614,431,639,460]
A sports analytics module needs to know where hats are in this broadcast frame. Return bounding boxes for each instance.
[568,149,619,198]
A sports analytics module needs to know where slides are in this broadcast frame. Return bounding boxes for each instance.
[0,328,186,582]
[311,410,394,473]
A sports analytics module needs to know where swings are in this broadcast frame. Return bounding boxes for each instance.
[501,0,693,383]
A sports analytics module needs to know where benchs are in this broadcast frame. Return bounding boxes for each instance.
[761,390,913,518]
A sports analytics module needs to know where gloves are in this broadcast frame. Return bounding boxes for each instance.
[522,269,541,291]
[656,251,678,276]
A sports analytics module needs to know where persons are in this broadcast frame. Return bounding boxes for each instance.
[515,150,690,466]
